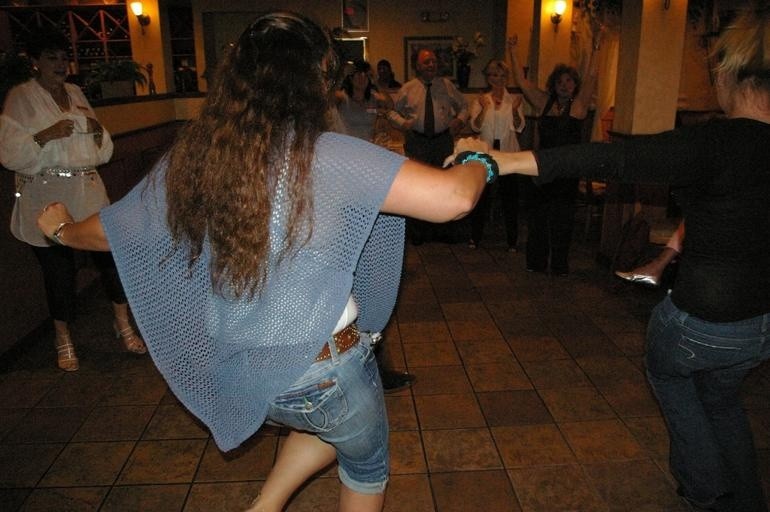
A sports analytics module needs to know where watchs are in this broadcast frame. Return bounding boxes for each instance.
[54,222,77,246]
[591,44,601,50]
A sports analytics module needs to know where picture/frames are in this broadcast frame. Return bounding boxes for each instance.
[341,0,370,31]
[405,37,460,84]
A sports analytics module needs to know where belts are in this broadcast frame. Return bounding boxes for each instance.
[315,323,361,360]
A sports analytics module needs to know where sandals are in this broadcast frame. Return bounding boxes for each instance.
[469,238,478,250]
[508,242,518,253]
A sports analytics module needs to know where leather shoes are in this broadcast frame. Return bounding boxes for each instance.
[615,270,662,287]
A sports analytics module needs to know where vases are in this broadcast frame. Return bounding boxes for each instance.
[98,80,137,97]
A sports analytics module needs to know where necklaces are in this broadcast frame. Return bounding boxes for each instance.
[554,95,573,114]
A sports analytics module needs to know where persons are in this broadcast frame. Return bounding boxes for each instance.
[39,13,491,512]
[332,58,387,143]
[374,59,403,90]
[443,1,770,512]
[508,20,608,277]
[388,49,472,245]
[615,214,686,291]
[0,29,147,372]
[469,60,526,253]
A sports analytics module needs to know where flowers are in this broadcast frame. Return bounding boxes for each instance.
[91,59,147,91]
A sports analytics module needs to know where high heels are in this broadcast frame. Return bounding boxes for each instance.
[113,315,147,354]
[55,330,80,371]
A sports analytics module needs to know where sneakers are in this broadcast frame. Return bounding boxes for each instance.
[526,255,547,270]
[550,260,569,278]
[382,371,416,393]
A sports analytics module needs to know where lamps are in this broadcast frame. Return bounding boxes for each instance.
[131,2,151,35]
[551,0,567,36]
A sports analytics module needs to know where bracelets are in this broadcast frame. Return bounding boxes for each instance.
[454,149,499,185]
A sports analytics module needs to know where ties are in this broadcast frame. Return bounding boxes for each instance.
[424,83,434,139]
